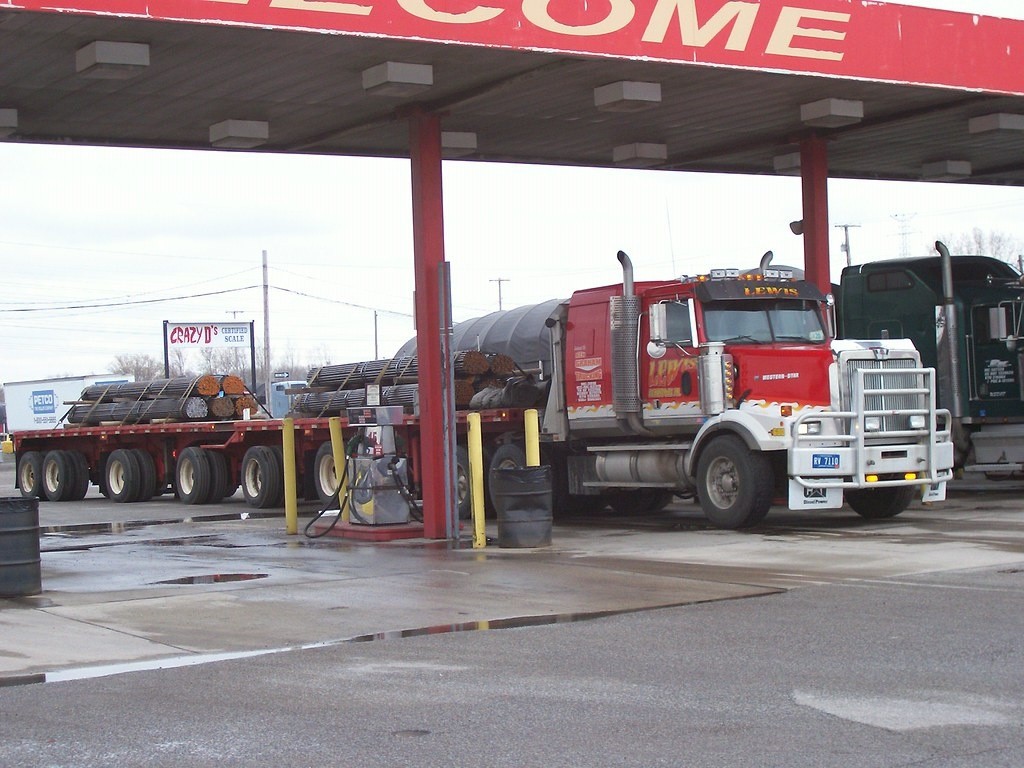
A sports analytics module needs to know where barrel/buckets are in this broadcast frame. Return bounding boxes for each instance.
[492,464,554,549]
[0,496,43,598]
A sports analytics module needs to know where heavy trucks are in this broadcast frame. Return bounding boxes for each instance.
[12,252,954,529]
[741,256,1024,481]
